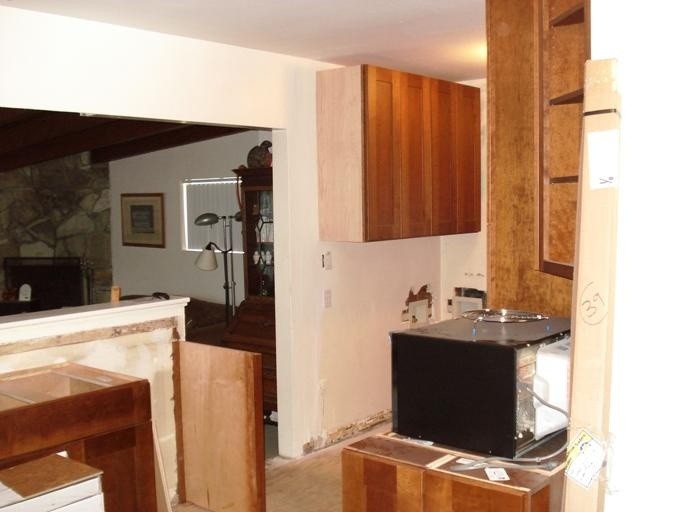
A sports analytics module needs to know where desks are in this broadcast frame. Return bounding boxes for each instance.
[339,437,563,511]
[1,359,159,511]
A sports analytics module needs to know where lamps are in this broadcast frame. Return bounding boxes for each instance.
[195,240,231,327]
[193,210,243,316]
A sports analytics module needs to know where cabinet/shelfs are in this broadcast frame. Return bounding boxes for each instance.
[316,64,482,243]
[538,0,592,282]
[226,166,278,423]
[0,455,109,512]
[0,295,266,511]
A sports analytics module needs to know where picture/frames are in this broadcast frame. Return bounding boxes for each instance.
[120,191,166,247]
